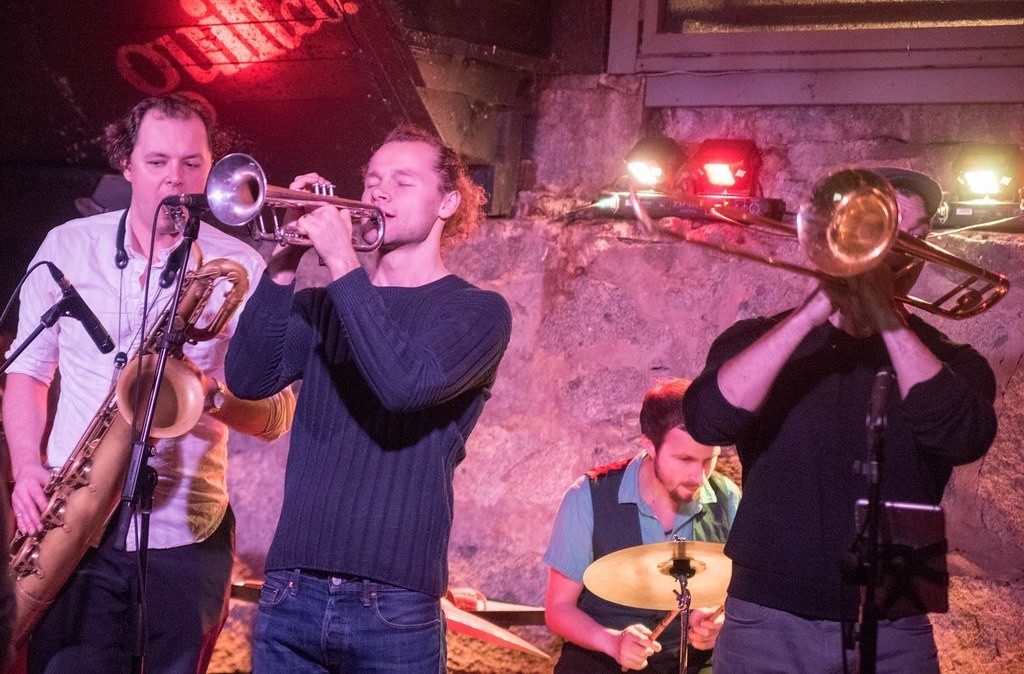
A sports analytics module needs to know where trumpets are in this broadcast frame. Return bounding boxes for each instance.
[204,152,386,266]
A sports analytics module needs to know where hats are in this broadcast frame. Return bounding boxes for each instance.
[873,165,941,219]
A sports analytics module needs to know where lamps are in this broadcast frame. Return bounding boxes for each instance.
[945,141,1024,232]
[616,135,786,223]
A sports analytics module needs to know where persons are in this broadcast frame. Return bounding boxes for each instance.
[226,123,511,674]
[684,164,998,674]
[0,93,296,674]
[543,377,742,674]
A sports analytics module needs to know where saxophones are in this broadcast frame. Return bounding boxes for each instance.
[4,197,252,662]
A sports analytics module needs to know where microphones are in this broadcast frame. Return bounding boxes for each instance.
[47,261,116,354]
[162,193,207,209]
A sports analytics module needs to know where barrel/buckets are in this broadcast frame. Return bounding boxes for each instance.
[445,587,487,611]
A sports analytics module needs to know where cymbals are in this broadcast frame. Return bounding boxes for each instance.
[439,597,551,659]
[582,539,731,611]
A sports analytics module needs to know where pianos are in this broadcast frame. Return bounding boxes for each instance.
[230,580,548,626]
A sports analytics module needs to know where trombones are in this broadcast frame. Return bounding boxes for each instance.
[627,168,1011,320]
